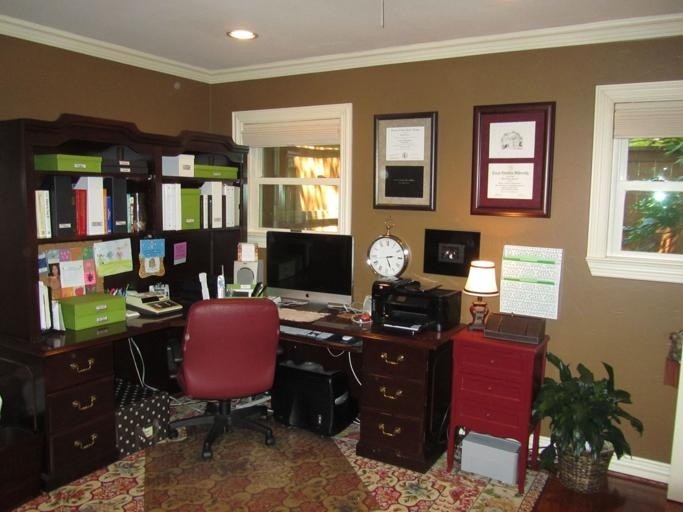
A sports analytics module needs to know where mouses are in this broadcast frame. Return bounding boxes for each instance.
[340,335,356,344]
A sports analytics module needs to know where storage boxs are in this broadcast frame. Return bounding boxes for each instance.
[115,376,171,460]
[194,165,239,179]
[35,153,102,173]
[58,294,127,331]
[182,189,200,229]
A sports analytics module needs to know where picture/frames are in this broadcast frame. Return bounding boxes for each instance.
[373,111,437,210]
[472,100,555,217]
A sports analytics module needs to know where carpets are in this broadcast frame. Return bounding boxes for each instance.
[10,409,555,511]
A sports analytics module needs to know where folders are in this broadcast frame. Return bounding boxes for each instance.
[72,177,104,235]
[42,174,73,237]
[106,177,127,233]
[198,181,222,228]
[221,183,234,227]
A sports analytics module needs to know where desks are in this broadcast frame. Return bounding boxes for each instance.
[0,296,187,490]
[273,298,461,474]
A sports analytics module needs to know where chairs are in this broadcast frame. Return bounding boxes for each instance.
[167,298,279,458]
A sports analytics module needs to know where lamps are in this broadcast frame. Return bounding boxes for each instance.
[463,260,500,331]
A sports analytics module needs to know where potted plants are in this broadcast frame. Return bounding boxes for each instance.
[528,354,644,493]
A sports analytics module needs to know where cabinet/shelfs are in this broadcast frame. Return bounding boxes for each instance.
[448,324,550,493]
[0,114,248,339]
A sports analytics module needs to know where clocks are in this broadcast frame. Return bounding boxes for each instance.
[365,223,409,280]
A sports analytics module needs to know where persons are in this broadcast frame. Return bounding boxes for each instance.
[47,264,60,276]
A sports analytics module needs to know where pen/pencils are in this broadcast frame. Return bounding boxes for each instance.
[104,283,130,297]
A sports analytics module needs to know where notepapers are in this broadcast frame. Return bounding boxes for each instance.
[83,260,97,292]
[59,285,85,297]
[46,246,94,264]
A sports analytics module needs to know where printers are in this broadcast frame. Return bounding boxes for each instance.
[371,288,462,337]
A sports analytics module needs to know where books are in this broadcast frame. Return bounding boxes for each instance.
[200,194,212,230]
[39,280,66,331]
[222,195,226,231]
[104,188,112,235]
[72,189,87,237]
[29,187,57,240]
[127,193,145,234]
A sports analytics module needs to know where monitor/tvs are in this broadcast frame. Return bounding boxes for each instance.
[266,231,355,312]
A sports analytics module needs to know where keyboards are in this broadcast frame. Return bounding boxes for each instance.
[280,325,335,341]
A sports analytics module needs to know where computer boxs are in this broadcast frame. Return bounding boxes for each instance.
[271,353,354,436]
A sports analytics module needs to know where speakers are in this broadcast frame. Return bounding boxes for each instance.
[234,260,264,285]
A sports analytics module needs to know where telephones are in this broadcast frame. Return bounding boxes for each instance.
[216,265,225,299]
